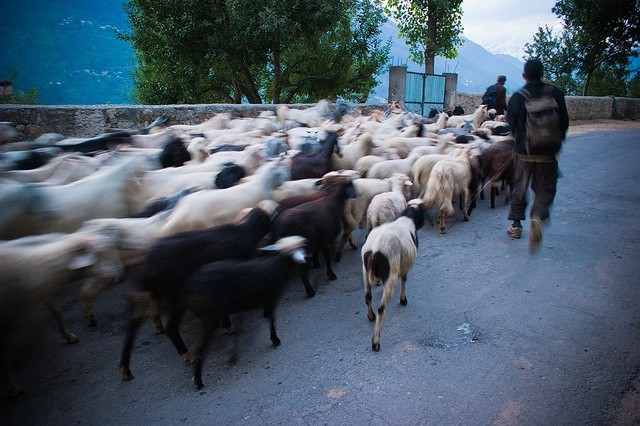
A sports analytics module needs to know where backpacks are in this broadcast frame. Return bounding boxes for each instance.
[483,85,498,108]
[515,88,562,156]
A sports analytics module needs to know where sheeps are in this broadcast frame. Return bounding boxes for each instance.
[367,173,415,229]
[270,174,358,300]
[184,235,316,391]
[118,197,285,382]
[426,141,483,229]
[0,96,525,387]
[360,197,427,353]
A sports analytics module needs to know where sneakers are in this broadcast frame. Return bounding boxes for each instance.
[530,215,542,242]
[507,225,522,238]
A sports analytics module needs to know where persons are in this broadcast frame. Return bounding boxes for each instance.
[481,76,508,115]
[507,59,569,249]
